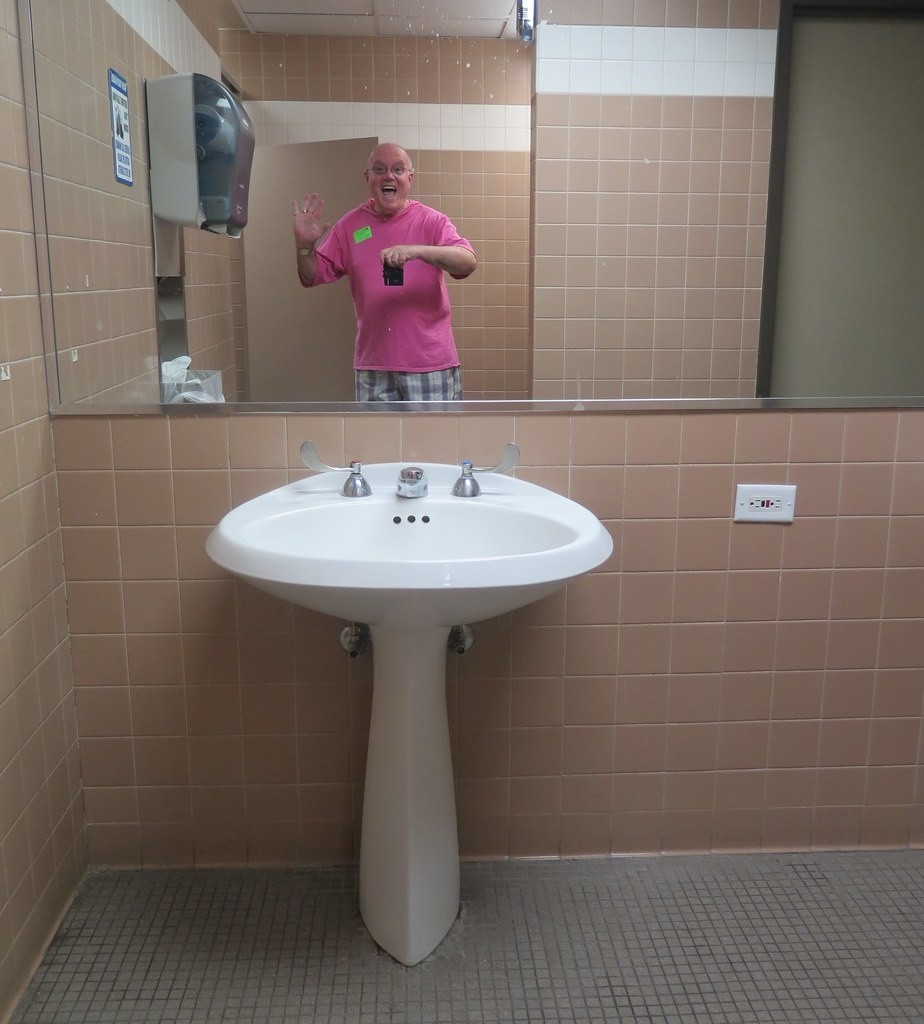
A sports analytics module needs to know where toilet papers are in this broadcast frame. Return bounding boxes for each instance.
[144,73,257,241]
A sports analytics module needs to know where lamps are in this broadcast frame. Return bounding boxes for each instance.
[517,0,536,42]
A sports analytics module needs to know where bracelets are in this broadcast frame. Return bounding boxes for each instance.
[296,246,315,256]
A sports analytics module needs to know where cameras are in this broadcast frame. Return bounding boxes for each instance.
[382,256,403,286]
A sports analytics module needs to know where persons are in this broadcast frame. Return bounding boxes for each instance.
[289,142,479,401]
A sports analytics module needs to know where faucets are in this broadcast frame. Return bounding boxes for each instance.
[395,466,430,499]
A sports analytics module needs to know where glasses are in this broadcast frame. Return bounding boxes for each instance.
[367,164,413,175]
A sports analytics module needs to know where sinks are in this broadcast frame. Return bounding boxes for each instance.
[202,459,618,629]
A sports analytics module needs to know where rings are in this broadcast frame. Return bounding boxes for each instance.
[302,209,308,213]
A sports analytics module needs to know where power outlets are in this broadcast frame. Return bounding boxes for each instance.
[733,484,797,523]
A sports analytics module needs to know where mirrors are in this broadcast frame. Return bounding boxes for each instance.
[16,0,923,417]
